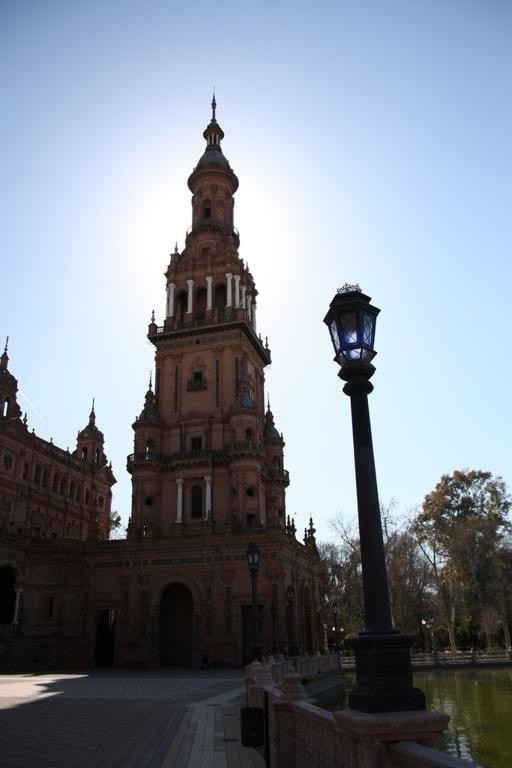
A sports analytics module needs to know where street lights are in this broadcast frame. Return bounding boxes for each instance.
[243,537,268,666]
[322,281,428,712]
[331,601,341,655]
[286,584,299,657]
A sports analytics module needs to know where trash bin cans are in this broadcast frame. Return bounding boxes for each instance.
[240,706,264,747]
